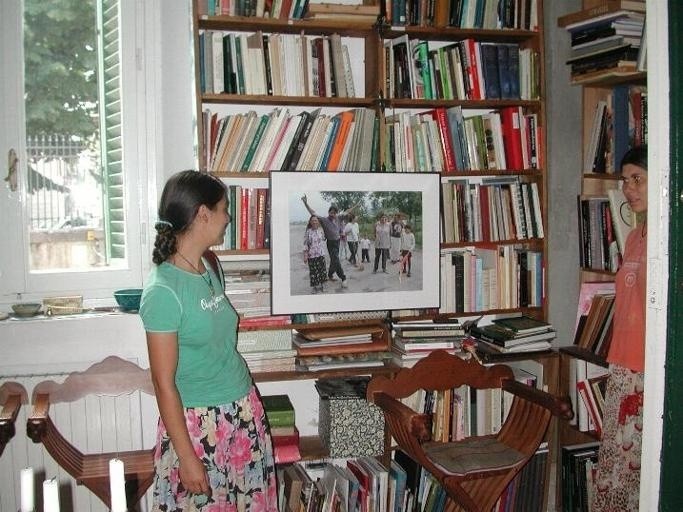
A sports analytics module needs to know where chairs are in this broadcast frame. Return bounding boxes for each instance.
[24,354,183,512]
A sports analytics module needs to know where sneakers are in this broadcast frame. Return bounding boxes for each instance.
[331,276,349,289]
[309,283,328,294]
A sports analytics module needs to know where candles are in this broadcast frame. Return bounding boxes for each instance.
[20,457,131,511]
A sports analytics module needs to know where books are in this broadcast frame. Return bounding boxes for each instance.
[577,374,607,435]
[384,35,540,101]
[401,370,538,443]
[261,394,301,464]
[387,105,544,173]
[200,30,355,99]
[202,108,379,171]
[224,269,297,373]
[440,176,544,244]
[583,85,649,176]
[391,0,537,32]
[196,0,305,20]
[392,243,542,317]
[574,282,617,359]
[274,448,547,512]
[558,0,646,76]
[576,180,637,274]
[562,441,597,512]
[294,317,558,370]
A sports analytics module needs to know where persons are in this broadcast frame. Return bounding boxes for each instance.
[138,167,278,511]
[301,195,415,289]
[592,144,648,512]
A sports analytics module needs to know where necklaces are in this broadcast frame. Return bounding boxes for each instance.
[176,250,214,300]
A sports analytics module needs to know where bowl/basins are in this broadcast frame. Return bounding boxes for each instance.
[112,288,143,310]
[12,302,41,318]
